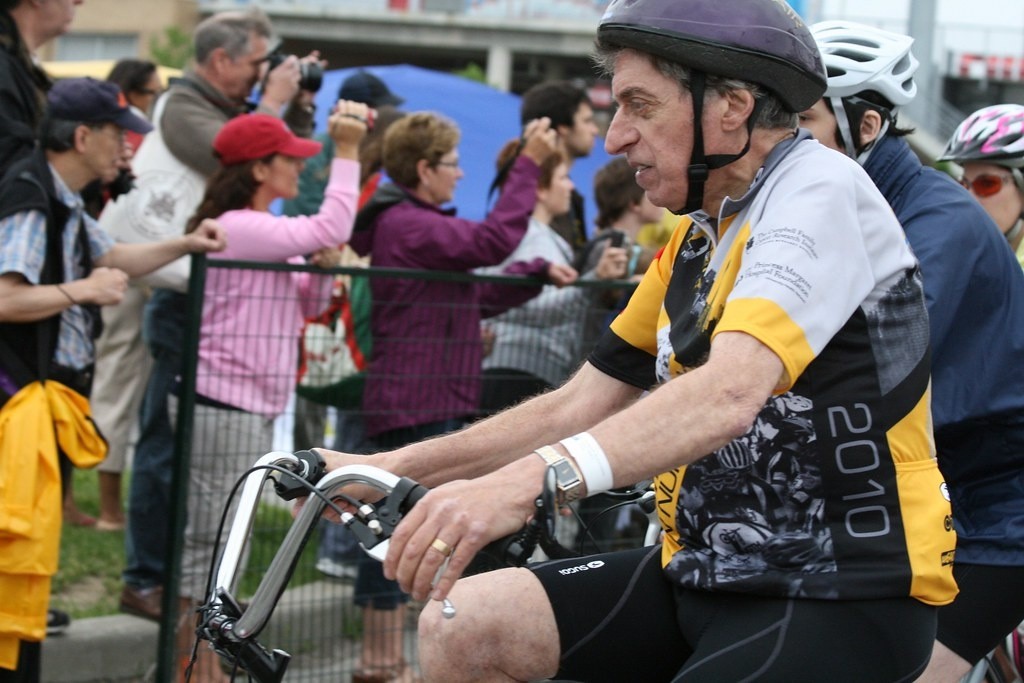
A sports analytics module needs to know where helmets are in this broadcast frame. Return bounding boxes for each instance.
[809,20,920,107]
[597,0,829,114]
[936,103,1024,169]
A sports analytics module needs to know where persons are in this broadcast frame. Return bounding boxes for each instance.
[268,1,960,681]
[1,0,673,682]
[935,102,1024,272]
[796,21,1023,683]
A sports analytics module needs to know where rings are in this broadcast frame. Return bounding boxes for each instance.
[431,538,451,557]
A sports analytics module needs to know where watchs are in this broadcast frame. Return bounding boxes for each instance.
[533,444,584,506]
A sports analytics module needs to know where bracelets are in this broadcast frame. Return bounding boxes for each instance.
[559,431,614,498]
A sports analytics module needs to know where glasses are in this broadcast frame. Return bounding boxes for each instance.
[958,175,1018,197]
[436,159,461,168]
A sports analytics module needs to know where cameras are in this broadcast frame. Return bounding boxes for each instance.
[108,166,136,203]
[261,56,322,93]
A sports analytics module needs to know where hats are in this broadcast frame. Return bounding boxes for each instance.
[339,71,404,110]
[211,113,323,168]
[47,77,155,134]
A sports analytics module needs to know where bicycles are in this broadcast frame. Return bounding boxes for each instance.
[184,448,1024,683]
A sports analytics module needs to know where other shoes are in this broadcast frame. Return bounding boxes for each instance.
[47,610,70,634]
[351,658,421,683]
[65,513,95,527]
[95,517,128,532]
[315,558,361,580]
[119,586,163,622]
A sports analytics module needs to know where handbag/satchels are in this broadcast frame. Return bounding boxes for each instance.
[94,86,210,295]
[294,246,374,411]
